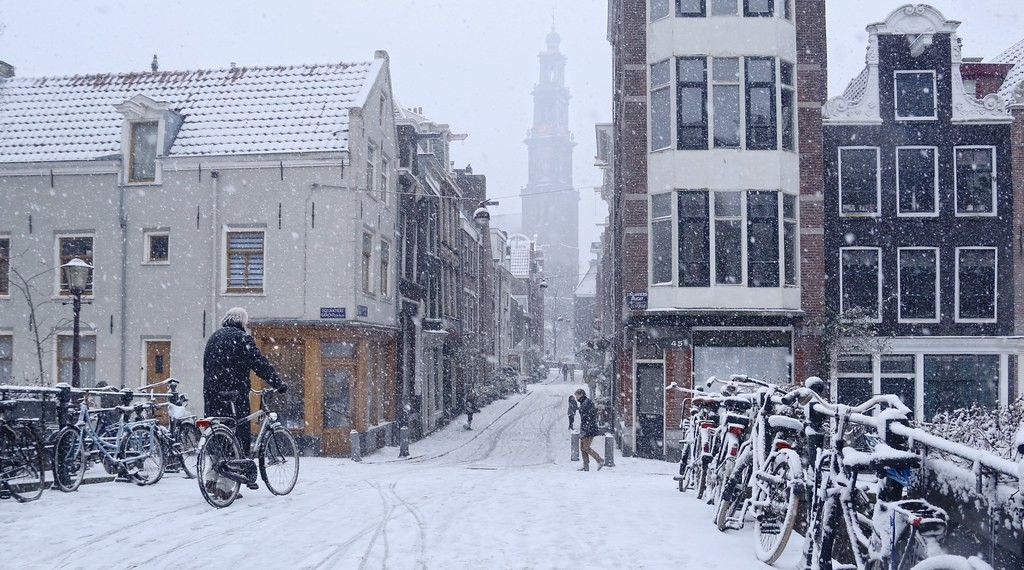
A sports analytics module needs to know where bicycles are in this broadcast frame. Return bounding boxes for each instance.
[0,378,205,503]
[665,374,950,570]
[195,388,299,509]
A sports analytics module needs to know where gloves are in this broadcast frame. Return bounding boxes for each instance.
[278,384,287,394]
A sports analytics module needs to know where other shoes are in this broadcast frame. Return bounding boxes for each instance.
[206,488,243,500]
[597,461,605,471]
[577,467,589,472]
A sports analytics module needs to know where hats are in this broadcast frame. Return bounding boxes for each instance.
[227,307,248,323]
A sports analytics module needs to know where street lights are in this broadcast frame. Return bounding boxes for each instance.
[399,296,421,457]
[596,340,616,434]
[60,258,94,411]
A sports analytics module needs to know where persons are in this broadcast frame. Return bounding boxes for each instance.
[558,360,575,381]
[203,307,288,500]
[568,389,604,471]
[463,393,480,431]
